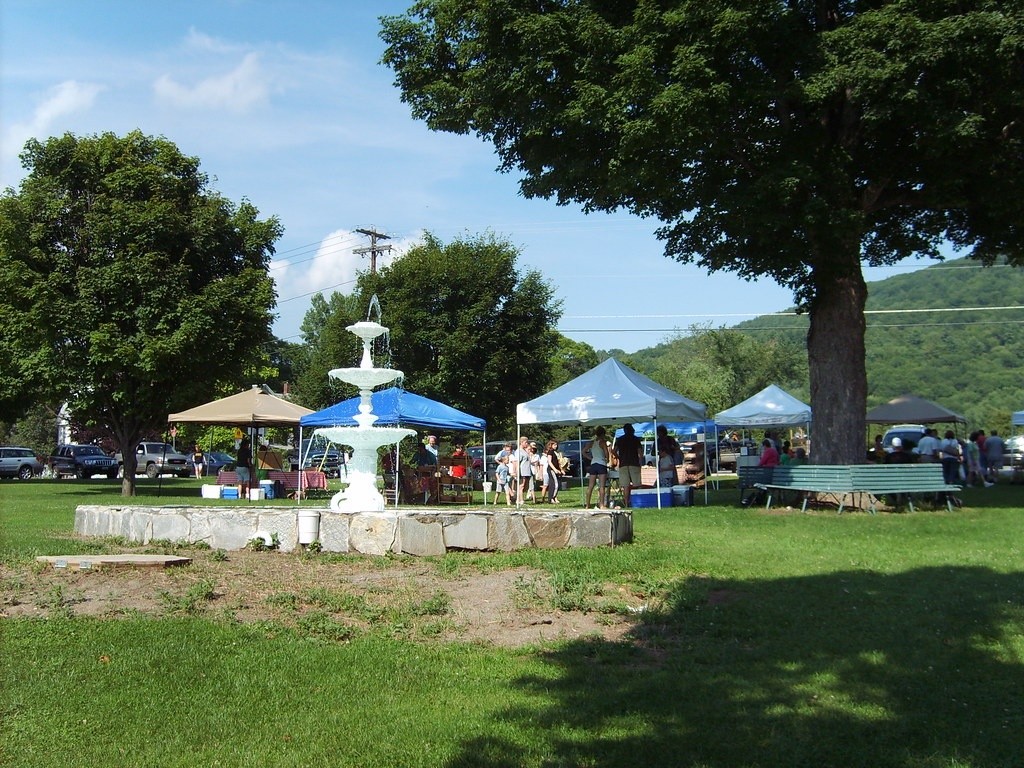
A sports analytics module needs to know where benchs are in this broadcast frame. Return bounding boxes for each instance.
[731,453,964,515]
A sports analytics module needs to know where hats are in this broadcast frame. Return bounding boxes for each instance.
[530,442,537,448]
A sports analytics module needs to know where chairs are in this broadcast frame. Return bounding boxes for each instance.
[381,473,403,505]
[585,471,627,507]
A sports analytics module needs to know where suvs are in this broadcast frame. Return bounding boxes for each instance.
[877,424,929,453]
[287,438,344,480]
[452,439,598,490]
[677,430,721,458]
[48,443,120,479]
[0,446,46,480]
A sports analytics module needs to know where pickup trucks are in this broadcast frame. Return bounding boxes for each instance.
[113,442,190,479]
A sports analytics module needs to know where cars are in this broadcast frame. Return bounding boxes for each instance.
[638,441,657,465]
[991,433,1024,467]
[186,450,237,476]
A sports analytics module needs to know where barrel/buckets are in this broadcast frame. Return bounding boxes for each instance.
[483,482,492,493]
[298,512,320,544]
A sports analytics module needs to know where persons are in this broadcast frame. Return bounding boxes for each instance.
[193,445,207,478]
[236,440,251,499]
[409,443,436,477]
[425,436,439,464]
[582,426,608,510]
[958,430,1005,488]
[731,432,739,441]
[449,445,467,477]
[382,446,402,474]
[494,436,573,505]
[874,435,883,452]
[758,440,780,467]
[760,430,807,464]
[613,423,643,505]
[253,445,267,480]
[651,426,679,487]
[888,428,963,483]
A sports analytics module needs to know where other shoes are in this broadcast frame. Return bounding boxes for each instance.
[550,499,560,503]
[985,482,994,487]
[967,484,975,488]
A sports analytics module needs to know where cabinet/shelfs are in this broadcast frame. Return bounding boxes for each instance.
[437,452,473,505]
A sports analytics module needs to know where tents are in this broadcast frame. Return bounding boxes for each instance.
[612,416,738,489]
[297,387,486,506]
[1011,410,1024,483]
[714,384,811,490]
[516,358,707,508]
[867,395,967,451]
[158,387,348,504]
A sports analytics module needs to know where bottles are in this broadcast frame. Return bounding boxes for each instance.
[268,490,272,500]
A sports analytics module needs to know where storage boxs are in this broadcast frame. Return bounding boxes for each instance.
[454,477,472,484]
[456,494,474,501]
[201,484,224,499]
[440,456,453,466]
[453,457,470,465]
[673,485,694,507]
[249,488,265,501]
[630,487,673,507]
[259,479,284,499]
[439,493,455,501]
[441,476,453,484]
[222,486,240,499]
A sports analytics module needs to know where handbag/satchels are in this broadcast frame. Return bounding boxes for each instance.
[943,438,958,456]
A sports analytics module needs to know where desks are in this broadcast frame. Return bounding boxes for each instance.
[216,471,328,499]
[641,467,689,486]
